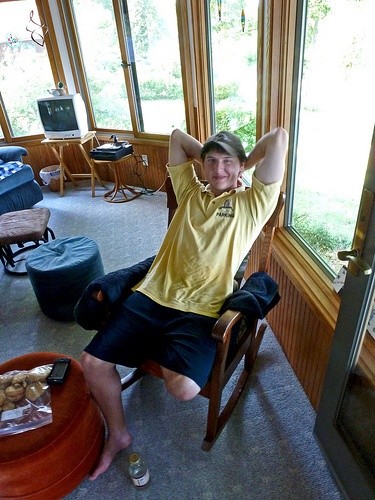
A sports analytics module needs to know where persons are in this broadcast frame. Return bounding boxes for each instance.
[79,126,288,480]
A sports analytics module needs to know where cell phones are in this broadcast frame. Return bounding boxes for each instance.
[46,358,71,384]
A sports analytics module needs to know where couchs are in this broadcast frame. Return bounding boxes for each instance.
[1,144,44,214]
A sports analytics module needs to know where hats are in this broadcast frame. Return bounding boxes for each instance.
[201,131,245,156]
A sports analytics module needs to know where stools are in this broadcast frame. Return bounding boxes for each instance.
[24,234,108,322]
[0,208,58,275]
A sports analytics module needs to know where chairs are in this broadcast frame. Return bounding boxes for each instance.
[72,179,287,449]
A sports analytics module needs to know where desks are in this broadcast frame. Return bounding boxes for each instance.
[40,130,106,197]
[94,154,141,202]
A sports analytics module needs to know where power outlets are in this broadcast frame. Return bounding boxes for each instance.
[142,155,148,166]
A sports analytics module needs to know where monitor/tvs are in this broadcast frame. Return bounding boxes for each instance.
[37,93,88,140]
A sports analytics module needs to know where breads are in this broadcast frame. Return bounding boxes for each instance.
[0,365,52,412]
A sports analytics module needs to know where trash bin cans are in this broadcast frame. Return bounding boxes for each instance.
[39,165,66,193]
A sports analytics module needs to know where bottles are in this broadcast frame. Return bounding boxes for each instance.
[127,453,149,490]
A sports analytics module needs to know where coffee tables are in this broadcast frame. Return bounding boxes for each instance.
[0,352,109,499]
